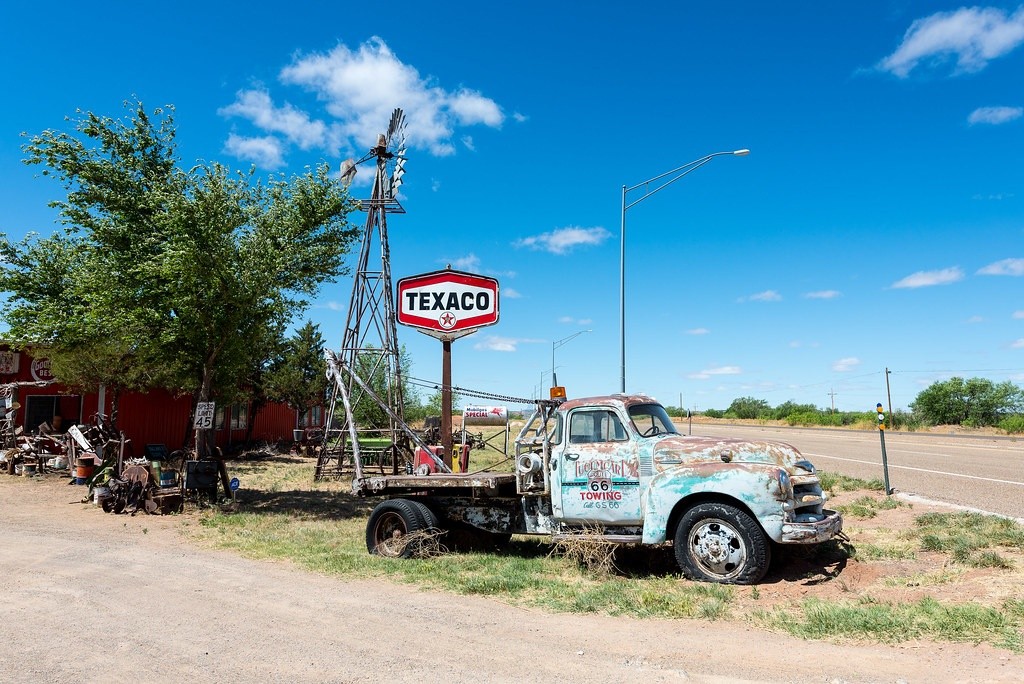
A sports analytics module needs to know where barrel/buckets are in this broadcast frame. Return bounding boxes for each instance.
[159,469,176,488]
[185,460,220,504]
[76,457,94,485]
[293,429,304,441]
[93,487,108,505]
[15,463,37,477]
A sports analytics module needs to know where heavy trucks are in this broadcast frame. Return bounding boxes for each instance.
[357,394,850,585]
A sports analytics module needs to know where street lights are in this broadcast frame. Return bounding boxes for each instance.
[552,329,592,386]
[531,365,568,399]
[619,149,750,393]
